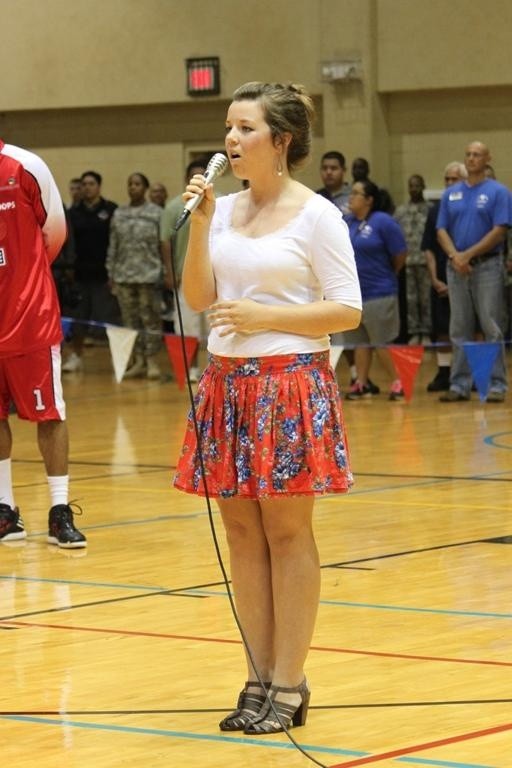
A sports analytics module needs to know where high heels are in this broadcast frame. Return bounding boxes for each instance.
[219,677,310,733]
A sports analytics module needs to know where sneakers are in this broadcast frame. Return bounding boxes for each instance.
[345,376,403,399]
[428,366,505,403]
[48,503,86,548]
[0,503,26,540]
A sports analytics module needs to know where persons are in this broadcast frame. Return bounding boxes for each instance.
[107,161,205,380]
[0,139,88,552]
[176,82,364,736]
[393,143,511,401]
[317,150,406,396]
[61,171,117,370]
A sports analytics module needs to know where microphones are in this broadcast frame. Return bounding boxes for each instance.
[173,152,227,233]
